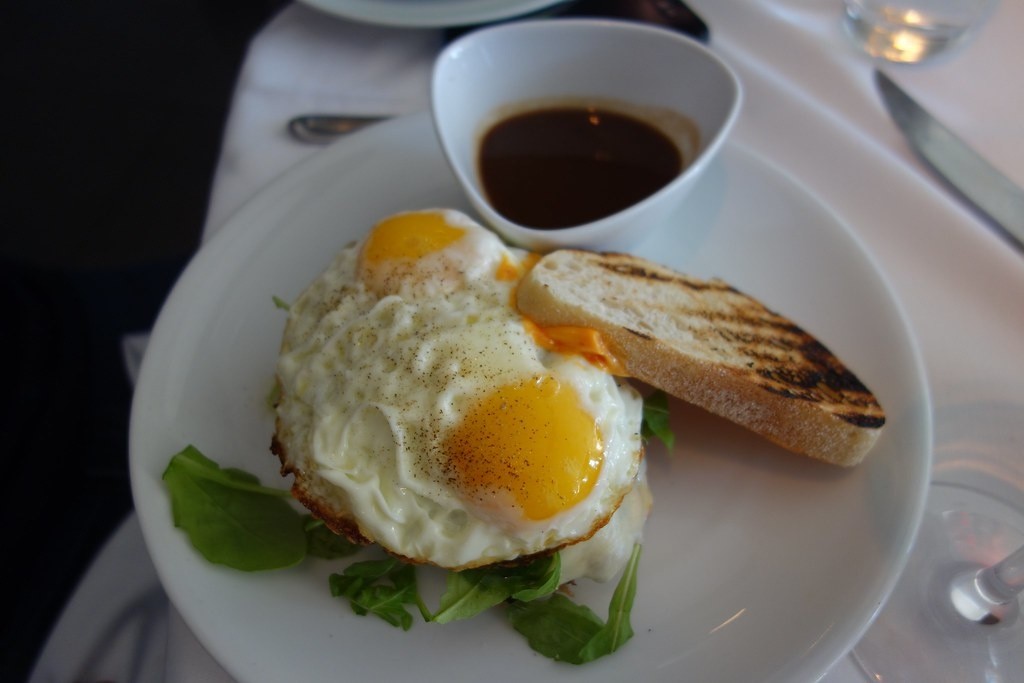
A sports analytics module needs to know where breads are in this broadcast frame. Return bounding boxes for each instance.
[515,253,884,469]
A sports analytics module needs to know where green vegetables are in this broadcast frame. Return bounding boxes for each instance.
[629,379,676,449]
[162,449,639,662]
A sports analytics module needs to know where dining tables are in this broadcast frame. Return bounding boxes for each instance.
[162,0,1024,683]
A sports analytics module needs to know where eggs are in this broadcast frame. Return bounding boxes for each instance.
[274,209,644,566]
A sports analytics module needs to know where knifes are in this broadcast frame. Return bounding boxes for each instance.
[874,64,1024,259]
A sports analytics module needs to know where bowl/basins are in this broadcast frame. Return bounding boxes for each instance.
[430,18,744,255]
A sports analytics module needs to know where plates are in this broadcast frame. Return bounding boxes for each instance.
[130,113,935,683]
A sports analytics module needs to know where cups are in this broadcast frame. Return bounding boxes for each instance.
[843,0,994,66]
